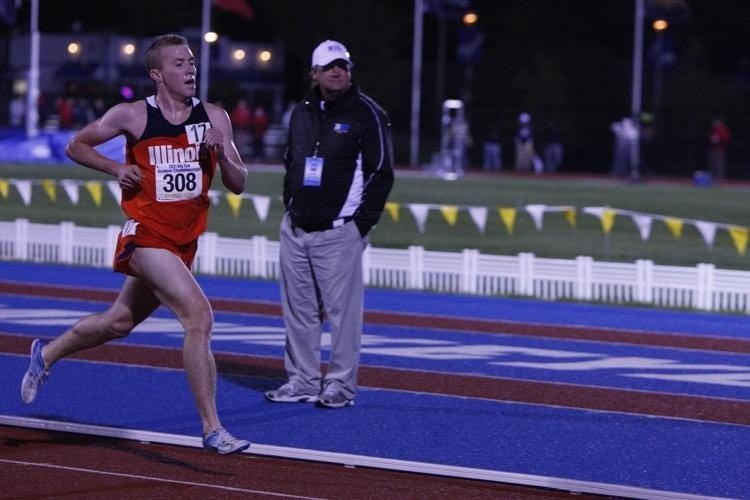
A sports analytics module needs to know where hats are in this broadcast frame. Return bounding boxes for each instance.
[312,39,354,68]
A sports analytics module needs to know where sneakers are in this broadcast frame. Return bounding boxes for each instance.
[20,338,51,405]
[264,381,355,407]
[203,426,250,455]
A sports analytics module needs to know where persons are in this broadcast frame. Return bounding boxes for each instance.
[263,40,395,408]
[710,114,731,180]
[20,35,251,455]
[8,97,298,158]
[483,113,563,174]
